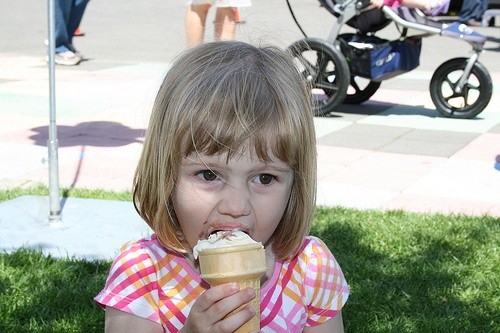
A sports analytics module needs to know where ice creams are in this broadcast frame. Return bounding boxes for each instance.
[193,230,266,333]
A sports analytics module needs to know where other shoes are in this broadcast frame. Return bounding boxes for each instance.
[72,49,89,60]
[55,50,81,65]
[440,0,451,14]
[427,0,448,16]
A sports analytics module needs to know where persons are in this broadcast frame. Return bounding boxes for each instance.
[370,0,449,23]
[186,0,252,49]
[94,41,349,333]
[46,0,89,65]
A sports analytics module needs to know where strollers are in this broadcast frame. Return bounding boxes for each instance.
[285,0,500,119]
